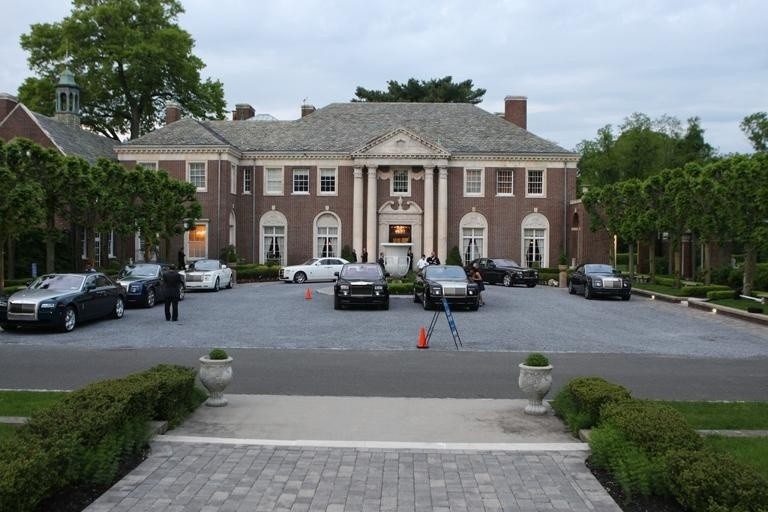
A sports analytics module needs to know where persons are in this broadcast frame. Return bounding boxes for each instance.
[469,267,485,307]
[407,249,414,268]
[427,251,440,265]
[377,252,385,271]
[416,254,429,271]
[84,263,96,272]
[162,263,181,321]
[178,246,187,270]
[472,262,484,283]
[361,247,367,263]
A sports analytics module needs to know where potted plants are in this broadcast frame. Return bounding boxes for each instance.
[198,351,234,407]
[226,244,239,284]
[518,351,555,417]
[557,253,568,289]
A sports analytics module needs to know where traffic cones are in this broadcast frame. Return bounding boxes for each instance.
[413,327,431,348]
[303,287,314,300]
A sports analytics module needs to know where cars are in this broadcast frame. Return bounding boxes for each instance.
[1,259,236,328]
[412,264,482,313]
[461,257,540,289]
[278,256,354,282]
[328,263,393,312]
[566,260,635,302]
